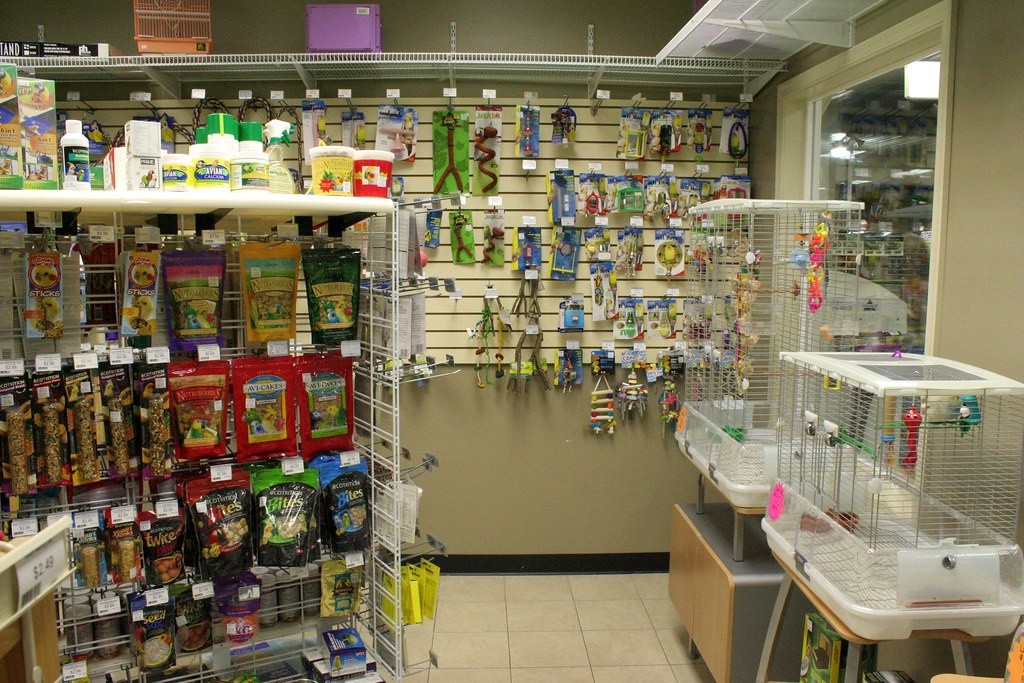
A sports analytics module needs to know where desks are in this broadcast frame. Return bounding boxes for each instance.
[756,551,990,683]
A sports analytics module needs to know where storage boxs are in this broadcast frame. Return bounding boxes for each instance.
[0,64,59,190]
[305,4,381,59]
[0,41,124,57]
[125,120,162,193]
[800,612,916,683]
[259,558,440,682]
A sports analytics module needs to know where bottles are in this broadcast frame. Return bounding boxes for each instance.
[191,112,264,157]
[60,120,93,190]
[57,555,339,670]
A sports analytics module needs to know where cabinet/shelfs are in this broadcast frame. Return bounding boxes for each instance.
[0,52,789,438]
[668,472,819,683]
[0,51,460,683]
[0,516,78,683]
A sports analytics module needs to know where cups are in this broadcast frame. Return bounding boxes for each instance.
[309,144,395,201]
